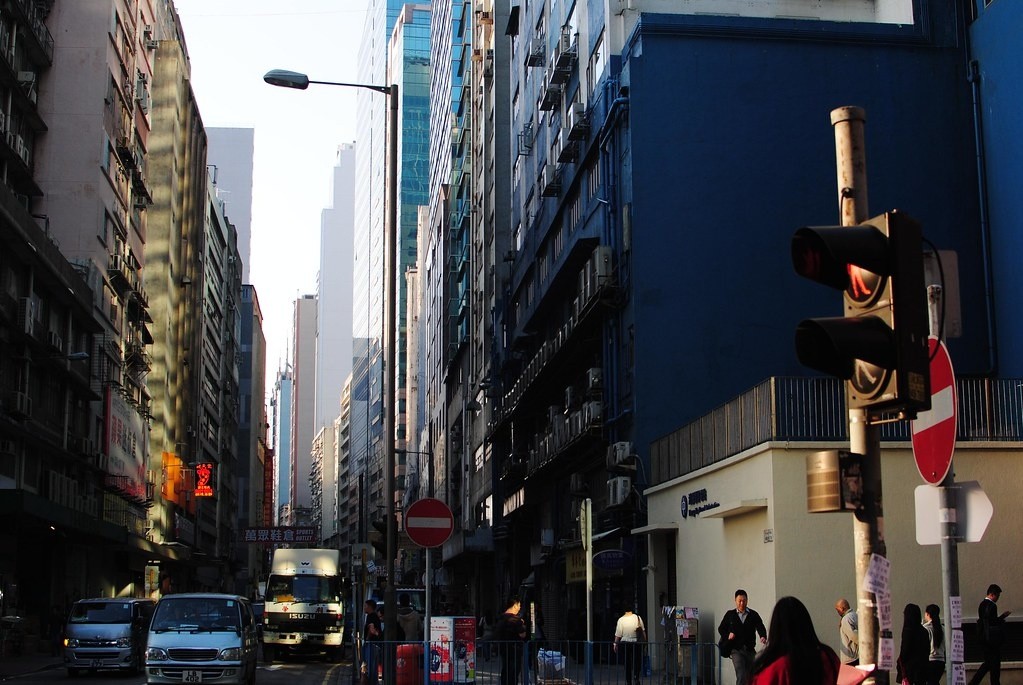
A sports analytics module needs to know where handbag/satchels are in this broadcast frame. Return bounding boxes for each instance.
[477,618,485,637]
[636,616,645,641]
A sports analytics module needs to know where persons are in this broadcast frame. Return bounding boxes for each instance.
[967,584,1011,685]
[494,595,527,685]
[478,615,494,662]
[922,604,945,685]
[166,605,186,626]
[835,599,859,667]
[362,600,386,685]
[745,596,840,685]
[525,605,545,671]
[718,590,767,685]
[613,605,646,685]
[895,603,930,685]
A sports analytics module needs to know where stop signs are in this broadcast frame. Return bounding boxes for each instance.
[909,334,959,486]
[404,498,455,549]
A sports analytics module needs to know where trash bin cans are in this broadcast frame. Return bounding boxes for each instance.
[396,643,424,685]
[0,615,25,658]
[663,606,699,678]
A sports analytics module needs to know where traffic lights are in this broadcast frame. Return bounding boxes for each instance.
[371,513,398,561]
[788,206,933,426]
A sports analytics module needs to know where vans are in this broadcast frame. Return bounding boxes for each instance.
[144,593,258,685]
[61,596,156,677]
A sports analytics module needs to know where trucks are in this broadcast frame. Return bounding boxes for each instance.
[254,545,348,663]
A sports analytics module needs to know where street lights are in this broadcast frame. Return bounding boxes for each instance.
[261,66,402,685]
[393,448,436,685]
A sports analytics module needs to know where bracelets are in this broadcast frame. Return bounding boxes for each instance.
[614,642,618,645]
